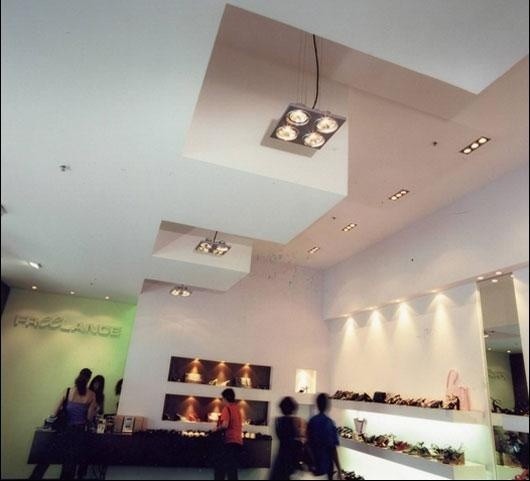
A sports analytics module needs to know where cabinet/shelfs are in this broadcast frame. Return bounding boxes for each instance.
[326,396,488,481]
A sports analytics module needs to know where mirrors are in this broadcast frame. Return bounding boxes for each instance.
[476,273,529,480]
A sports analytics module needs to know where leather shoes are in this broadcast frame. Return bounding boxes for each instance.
[344,471,364,481]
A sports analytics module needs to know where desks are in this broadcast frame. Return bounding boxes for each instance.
[27,429,271,480]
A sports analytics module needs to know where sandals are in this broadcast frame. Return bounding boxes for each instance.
[337,426,464,465]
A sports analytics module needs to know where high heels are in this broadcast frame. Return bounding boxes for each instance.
[328,391,460,410]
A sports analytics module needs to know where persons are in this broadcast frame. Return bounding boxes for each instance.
[87,374,106,415]
[208,389,244,481]
[266,397,308,481]
[304,392,344,481]
[28,368,97,480]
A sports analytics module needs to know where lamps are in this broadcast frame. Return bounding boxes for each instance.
[193,239,231,255]
[169,286,192,297]
[261,103,345,158]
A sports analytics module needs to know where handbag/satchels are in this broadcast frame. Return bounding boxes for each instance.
[207,428,225,446]
[51,388,70,434]
